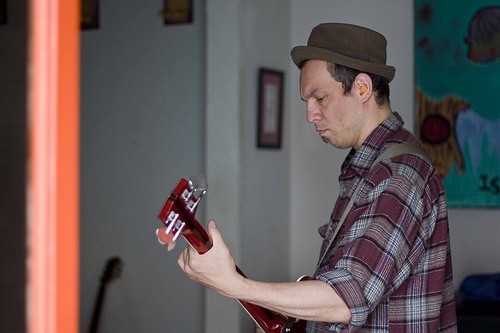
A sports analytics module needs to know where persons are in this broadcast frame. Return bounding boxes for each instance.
[178,22,457,333]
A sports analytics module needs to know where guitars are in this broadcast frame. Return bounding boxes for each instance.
[156,176,317,333]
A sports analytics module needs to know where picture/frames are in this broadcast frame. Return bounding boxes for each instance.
[164,0,194,26]
[81,0,101,31]
[256,67,285,150]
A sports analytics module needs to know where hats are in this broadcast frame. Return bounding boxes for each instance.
[290,22,396,82]
